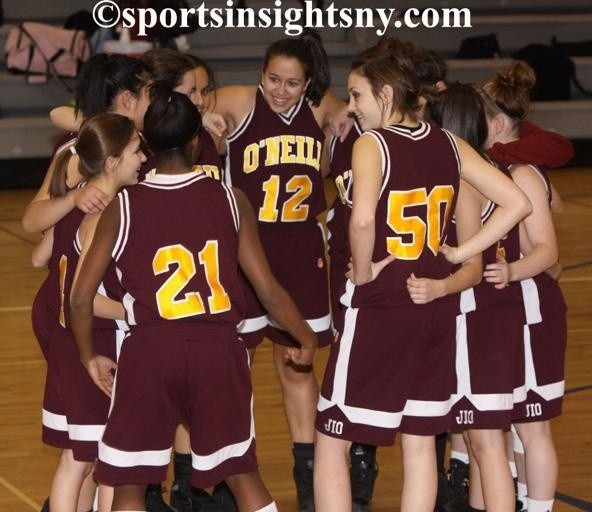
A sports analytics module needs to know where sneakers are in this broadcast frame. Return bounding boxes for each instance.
[292,459,315,512]
[145,492,174,512]
[434,460,469,512]
[171,482,240,512]
[348,442,376,502]
